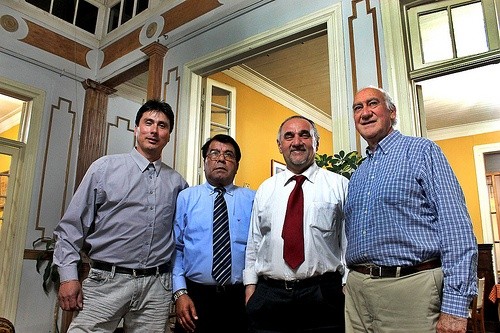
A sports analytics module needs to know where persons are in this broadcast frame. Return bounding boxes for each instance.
[54,98,189,333]
[172,134,256,333]
[343,85,478,333]
[243,116,349,333]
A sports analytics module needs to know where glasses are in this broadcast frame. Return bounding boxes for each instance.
[205,150,235,160]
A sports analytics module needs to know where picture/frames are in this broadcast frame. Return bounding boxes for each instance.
[271,159,286,176]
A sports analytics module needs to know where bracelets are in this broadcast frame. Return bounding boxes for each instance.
[174,289,188,301]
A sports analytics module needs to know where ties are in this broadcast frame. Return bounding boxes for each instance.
[281,175,308,269]
[211,186,231,288]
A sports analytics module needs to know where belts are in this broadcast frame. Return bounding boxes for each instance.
[91,258,171,275]
[348,257,442,280]
[262,271,342,291]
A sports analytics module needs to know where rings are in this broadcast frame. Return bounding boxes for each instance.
[178,318,182,325]
